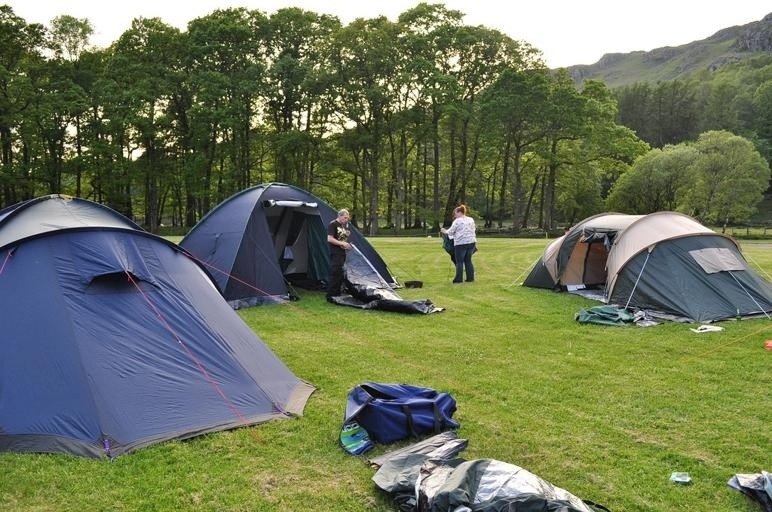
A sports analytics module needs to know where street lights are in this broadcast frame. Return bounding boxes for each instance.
[339,384,460,456]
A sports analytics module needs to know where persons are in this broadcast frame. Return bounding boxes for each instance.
[325,208,352,303]
[440,205,477,284]
